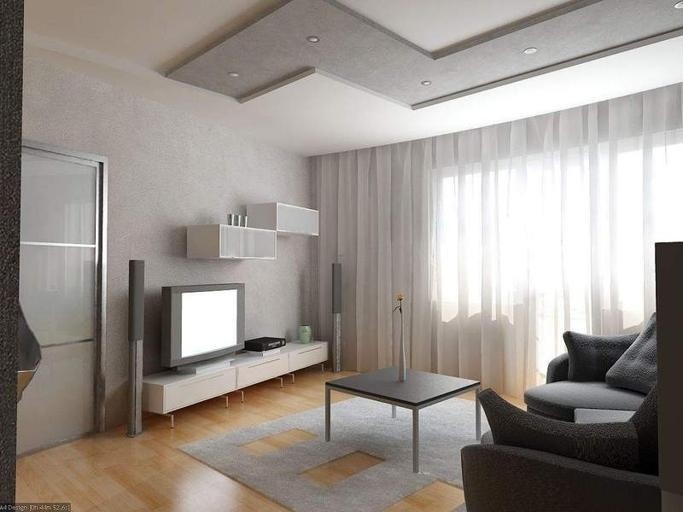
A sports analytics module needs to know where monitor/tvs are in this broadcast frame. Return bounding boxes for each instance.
[161,283,245,367]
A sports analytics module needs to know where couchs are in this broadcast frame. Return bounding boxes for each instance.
[459,331,660,512]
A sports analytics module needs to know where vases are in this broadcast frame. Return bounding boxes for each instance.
[397,311,406,382]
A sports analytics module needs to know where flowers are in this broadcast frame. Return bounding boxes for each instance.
[391,294,406,313]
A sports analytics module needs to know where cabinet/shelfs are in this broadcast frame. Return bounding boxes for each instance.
[141,342,328,429]
[184,200,320,261]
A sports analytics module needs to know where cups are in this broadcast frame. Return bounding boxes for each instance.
[227,214,248,227]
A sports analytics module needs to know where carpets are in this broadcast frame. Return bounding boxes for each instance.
[175,396,491,512]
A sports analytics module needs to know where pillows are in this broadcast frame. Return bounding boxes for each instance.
[605,312,658,394]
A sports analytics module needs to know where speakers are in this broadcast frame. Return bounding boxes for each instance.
[128,258,144,437]
[331,262,342,372]
